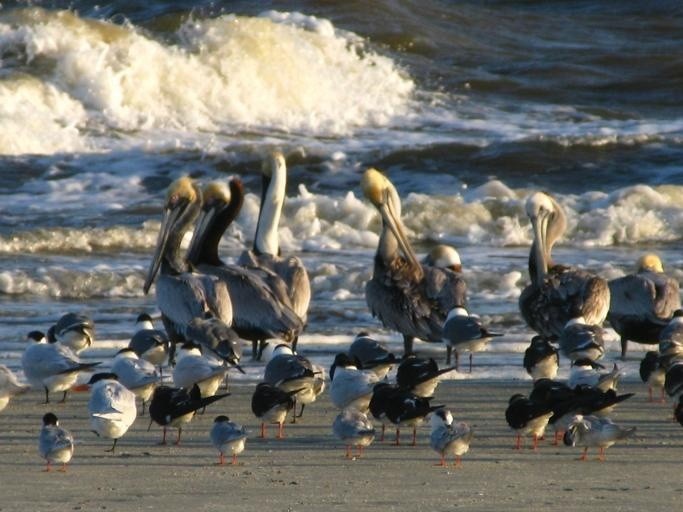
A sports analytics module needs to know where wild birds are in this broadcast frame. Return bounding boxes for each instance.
[329,166,504,470]
[1,308,99,473]
[86,148,325,468]
[506,189,683,460]
